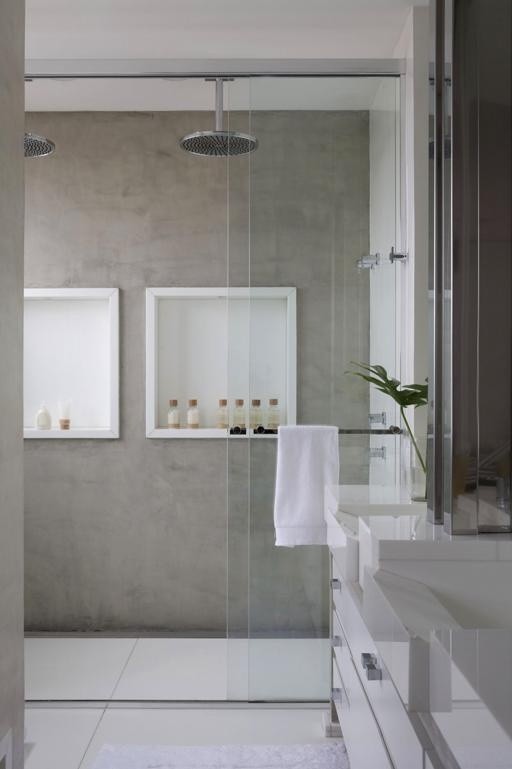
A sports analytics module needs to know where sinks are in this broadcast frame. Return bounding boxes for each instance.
[360,564,512,715]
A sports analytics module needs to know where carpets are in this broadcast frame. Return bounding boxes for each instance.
[89,740,351,768]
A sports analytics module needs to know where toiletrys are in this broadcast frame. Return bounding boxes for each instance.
[58,402,71,430]
[168,399,179,430]
[35,401,52,430]
[187,400,200,429]
[268,399,279,427]
[220,399,229,429]
[234,400,244,429]
[250,399,262,430]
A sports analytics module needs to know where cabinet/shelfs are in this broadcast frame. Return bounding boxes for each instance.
[24,286,121,440]
[144,286,297,439]
[330,555,436,769]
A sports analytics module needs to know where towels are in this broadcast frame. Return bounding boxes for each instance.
[274,425,349,549]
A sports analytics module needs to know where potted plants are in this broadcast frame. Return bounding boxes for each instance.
[342,358,428,502]
